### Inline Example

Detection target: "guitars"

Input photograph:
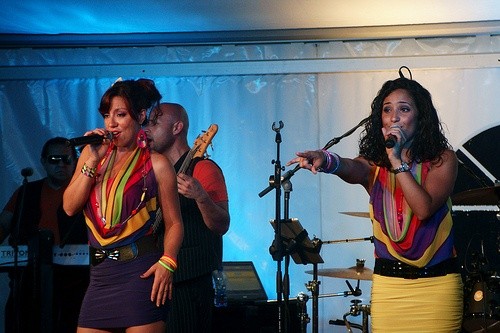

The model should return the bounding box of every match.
[153,123,219,234]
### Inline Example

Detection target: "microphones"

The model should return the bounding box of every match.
[21,167,33,176]
[384,125,401,148]
[68,131,112,147]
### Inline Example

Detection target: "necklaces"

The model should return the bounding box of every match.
[96,136,147,228]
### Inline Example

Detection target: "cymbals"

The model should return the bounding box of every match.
[338,211,370,218]
[451,186,500,205]
[303,265,373,280]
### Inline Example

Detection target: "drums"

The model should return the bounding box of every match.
[462,271,500,333]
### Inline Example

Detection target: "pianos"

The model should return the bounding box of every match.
[0,244,90,333]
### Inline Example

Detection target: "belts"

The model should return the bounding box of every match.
[373,258,459,280]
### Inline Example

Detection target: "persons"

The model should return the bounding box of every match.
[142,102,231,333]
[63,77,183,333]
[287,77,465,333]
[1,137,91,333]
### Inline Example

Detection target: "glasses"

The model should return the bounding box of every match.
[46,154,73,165]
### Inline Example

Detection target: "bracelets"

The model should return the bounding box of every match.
[158,254,178,273]
[80,163,97,178]
[319,149,340,174]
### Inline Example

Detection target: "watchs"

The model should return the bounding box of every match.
[392,161,412,174]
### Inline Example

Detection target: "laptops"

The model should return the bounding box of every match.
[222,261,297,304]
